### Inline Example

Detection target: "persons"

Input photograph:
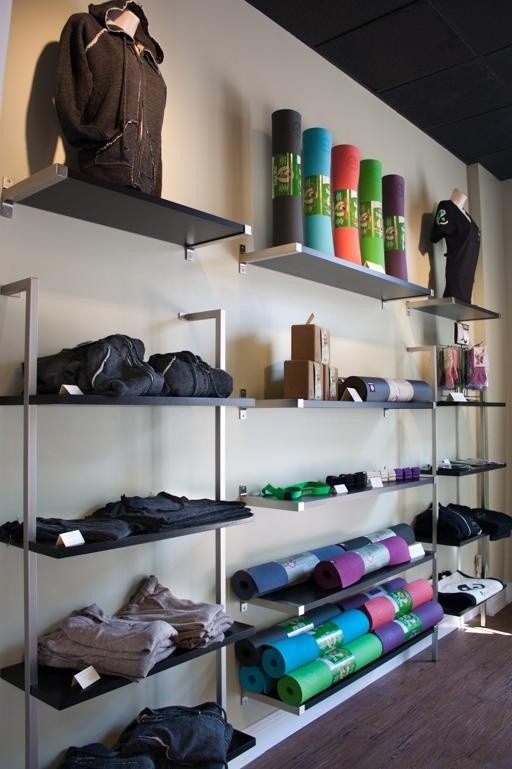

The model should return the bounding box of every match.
[53,2,165,199]
[428,189,480,304]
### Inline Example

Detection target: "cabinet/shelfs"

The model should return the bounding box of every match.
[1,273,256,768]
[405,343,508,664]
[239,388,441,720]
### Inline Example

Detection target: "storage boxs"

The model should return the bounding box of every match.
[283,359,322,402]
[322,365,340,401]
[290,323,331,364]
